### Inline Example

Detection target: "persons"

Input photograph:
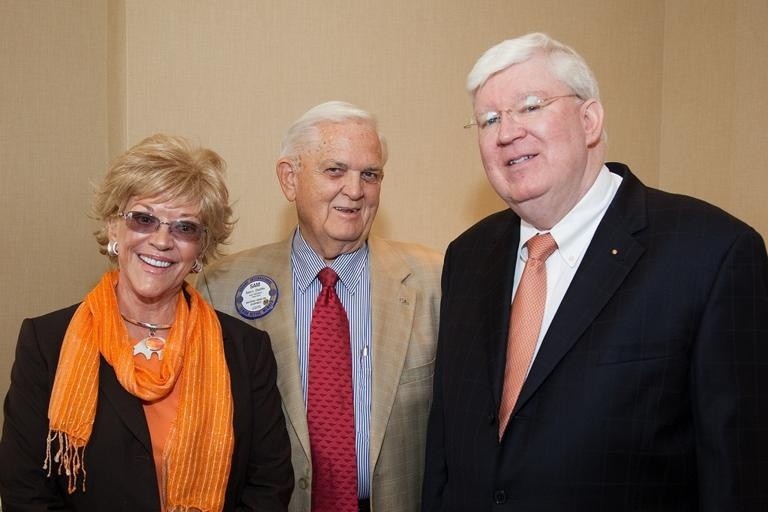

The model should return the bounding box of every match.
[0,133,295,512]
[195,100,445,512]
[421,30,768,512]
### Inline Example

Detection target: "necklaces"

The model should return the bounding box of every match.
[120,310,172,361]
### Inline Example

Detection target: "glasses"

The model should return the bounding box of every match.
[464,94,576,136]
[118,210,206,242]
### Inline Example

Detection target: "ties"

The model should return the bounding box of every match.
[498,234,558,441]
[306,268,359,512]
